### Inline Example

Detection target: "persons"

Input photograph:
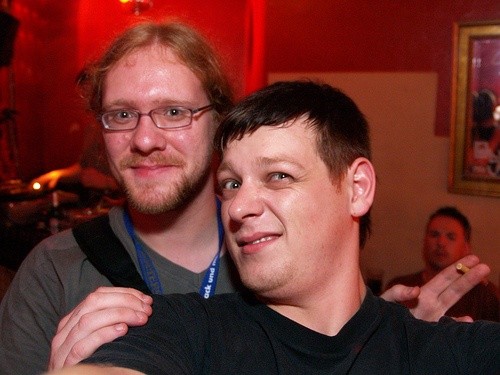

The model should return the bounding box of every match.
[44,80,500,375]
[0,23,492,374]
[385,206,500,323]
[463,87,500,182]
[27,61,129,235]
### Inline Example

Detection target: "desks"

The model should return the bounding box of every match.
[10,189,78,226]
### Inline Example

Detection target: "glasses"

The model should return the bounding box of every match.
[101,103,213,129]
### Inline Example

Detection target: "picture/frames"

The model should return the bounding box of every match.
[446,15,500,199]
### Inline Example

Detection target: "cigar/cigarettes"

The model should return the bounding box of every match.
[456,262,489,287]
